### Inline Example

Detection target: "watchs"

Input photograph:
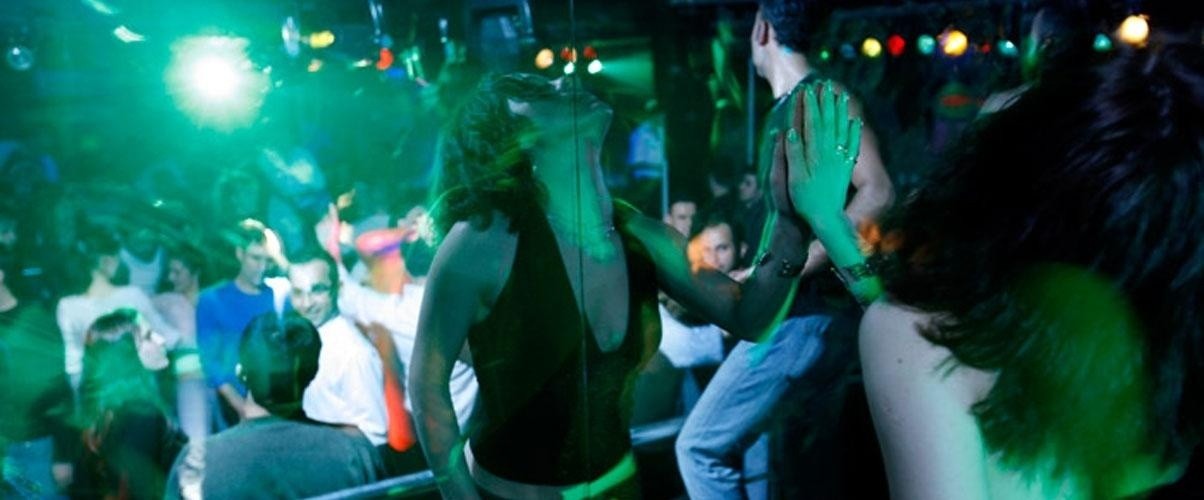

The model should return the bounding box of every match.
[756,249,805,278]
[830,244,892,285]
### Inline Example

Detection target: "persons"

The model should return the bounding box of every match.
[406,67,810,499]
[786,0,1203,500]
[632,154,769,424]
[1,3,480,499]
[673,0,895,499]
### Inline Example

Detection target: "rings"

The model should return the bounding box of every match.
[837,144,848,155]
[846,155,858,164]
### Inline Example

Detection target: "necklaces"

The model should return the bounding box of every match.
[547,210,616,246]
